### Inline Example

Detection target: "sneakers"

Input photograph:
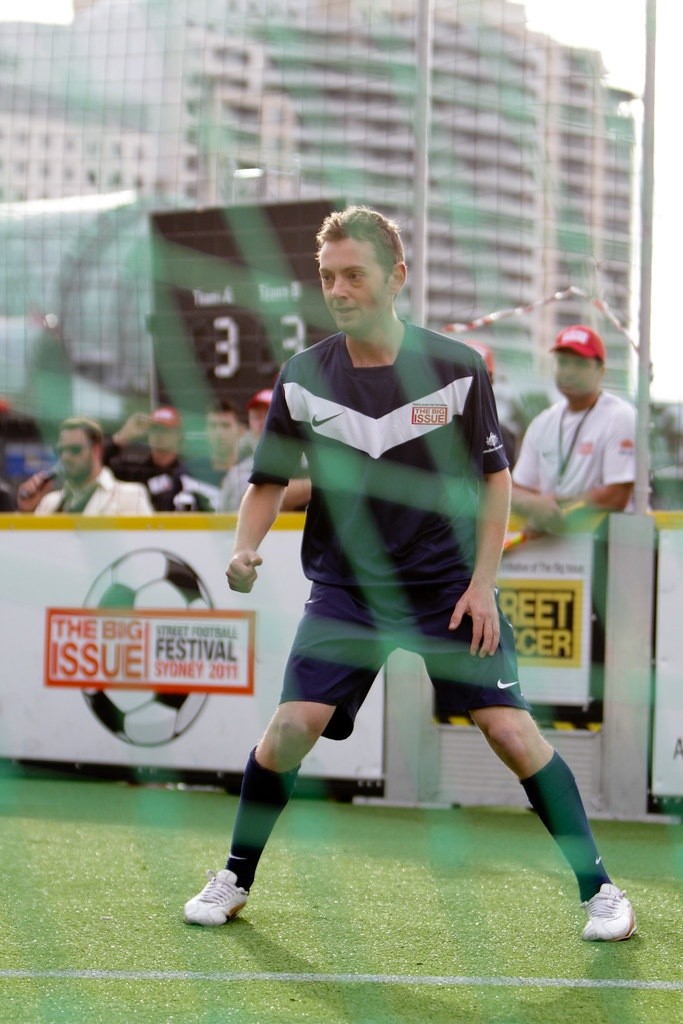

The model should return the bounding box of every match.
[183,870,248,927]
[580,883,637,941]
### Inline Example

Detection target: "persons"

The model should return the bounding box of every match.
[188,398,241,511]
[17,419,152,513]
[510,326,653,533]
[100,408,200,511]
[464,341,516,470]
[221,391,311,511]
[183,209,637,941]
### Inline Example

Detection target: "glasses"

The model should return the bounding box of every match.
[52,443,93,456]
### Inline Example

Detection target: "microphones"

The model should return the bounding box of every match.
[20,463,66,501]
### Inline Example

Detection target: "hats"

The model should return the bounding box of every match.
[467,340,494,373]
[248,390,272,407]
[150,408,180,428]
[549,326,605,364]
[113,412,150,445]
[7,448,57,474]
